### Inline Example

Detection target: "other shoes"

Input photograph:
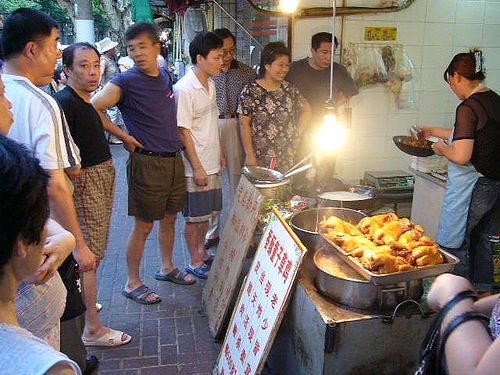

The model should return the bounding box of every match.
[204,236,219,250]
[108,138,124,144]
[83,355,98,375]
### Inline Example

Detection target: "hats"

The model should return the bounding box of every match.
[95,37,119,55]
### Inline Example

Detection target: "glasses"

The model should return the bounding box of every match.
[222,47,237,57]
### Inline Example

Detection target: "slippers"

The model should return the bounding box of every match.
[185,252,215,279]
[121,283,161,305]
[154,267,197,286]
[81,328,132,347]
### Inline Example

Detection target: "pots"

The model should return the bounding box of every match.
[254,176,293,202]
[288,207,368,255]
[312,245,424,315]
[316,184,378,212]
[392,135,435,157]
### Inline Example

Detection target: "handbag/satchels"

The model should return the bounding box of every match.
[354,47,415,90]
[411,289,491,375]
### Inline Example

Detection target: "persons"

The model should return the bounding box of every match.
[238,42,312,174]
[428,273,500,375]
[0,8,99,375]
[0,133,81,375]
[90,22,196,303]
[172,31,224,278]
[205,28,258,248]
[417,47,500,290]
[54,42,132,346]
[284,32,359,184]
[42,70,67,94]
[0,74,76,350]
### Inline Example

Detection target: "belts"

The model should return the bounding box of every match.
[219,112,239,119]
[134,145,182,158]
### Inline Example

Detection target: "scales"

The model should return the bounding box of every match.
[365,169,415,192]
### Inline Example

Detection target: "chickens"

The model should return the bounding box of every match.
[319,213,444,271]
